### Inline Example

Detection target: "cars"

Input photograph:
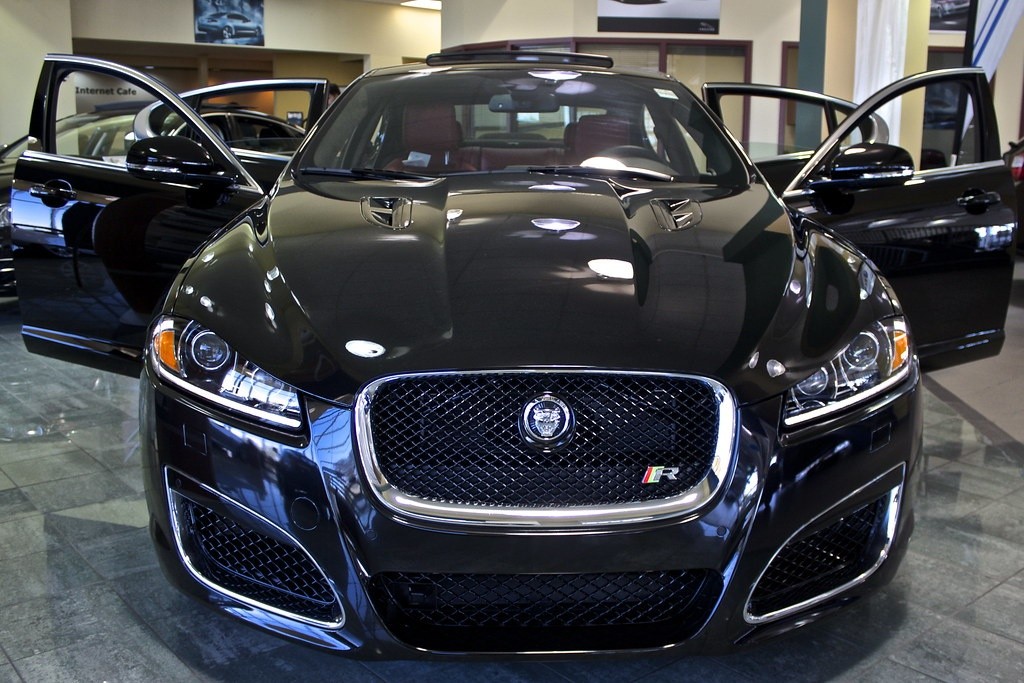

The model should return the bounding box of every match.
[999,136,1024,210]
[9,51,1020,659]
[0,98,319,306]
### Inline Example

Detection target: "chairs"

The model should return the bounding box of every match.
[379,96,475,172]
[574,113,640,164]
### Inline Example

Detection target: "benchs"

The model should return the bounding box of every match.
[453,121,577,166]
